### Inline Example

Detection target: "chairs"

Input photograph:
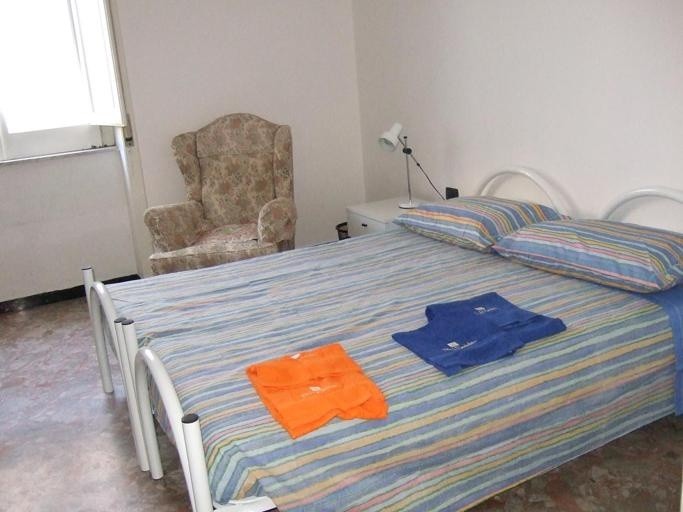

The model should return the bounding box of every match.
[144,113,296,275]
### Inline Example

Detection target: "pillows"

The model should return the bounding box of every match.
[392,195,573,254]
[490,218,683,294]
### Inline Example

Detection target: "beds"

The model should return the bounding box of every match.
[80,167,683,512]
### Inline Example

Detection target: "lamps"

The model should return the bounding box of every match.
[380,122,446,209]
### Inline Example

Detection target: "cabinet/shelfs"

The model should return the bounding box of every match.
[346,196,431,239]
